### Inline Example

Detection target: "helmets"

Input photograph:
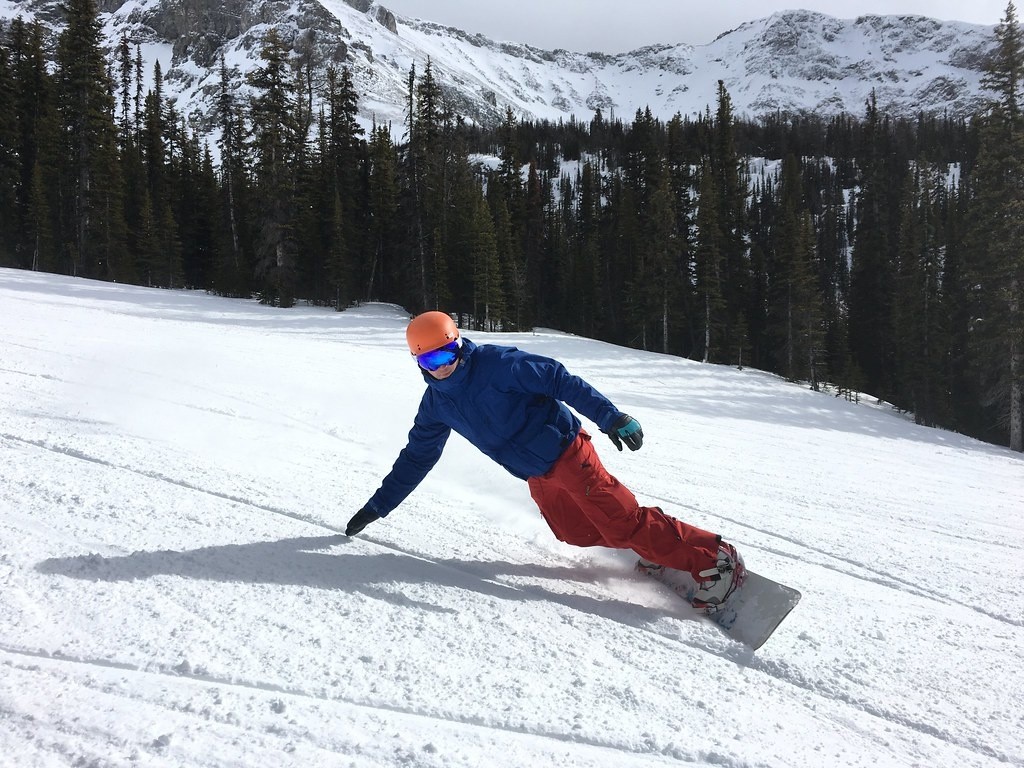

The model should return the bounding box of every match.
[407,311,459,357]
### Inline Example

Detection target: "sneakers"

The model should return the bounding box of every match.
[633,557,668,577]
[691,543,744,614]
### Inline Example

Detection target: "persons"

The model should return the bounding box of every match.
[345,311,747,614]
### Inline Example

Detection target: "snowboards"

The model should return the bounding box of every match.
[633,539,802,651]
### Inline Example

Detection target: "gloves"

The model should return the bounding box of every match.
[608,414,644,452]
[345,506,380,536]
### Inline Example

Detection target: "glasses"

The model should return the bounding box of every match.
[417,342,460,371]
